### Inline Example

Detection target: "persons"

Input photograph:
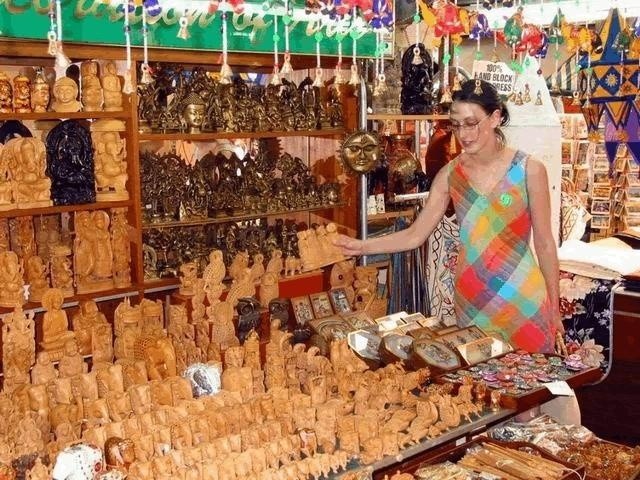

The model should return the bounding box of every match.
[331,77,564,424]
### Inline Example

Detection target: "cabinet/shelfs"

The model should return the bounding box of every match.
[1,38,360,373]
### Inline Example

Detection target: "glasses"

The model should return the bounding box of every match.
[447,113,492,130]
